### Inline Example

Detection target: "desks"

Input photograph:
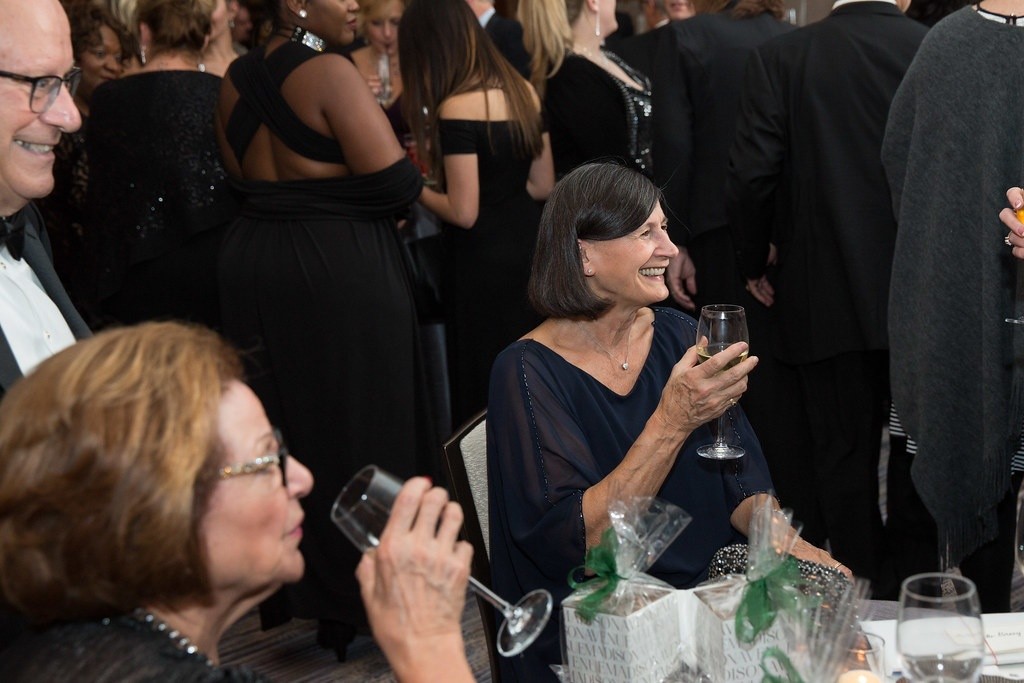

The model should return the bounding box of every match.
[858,601,1022,683]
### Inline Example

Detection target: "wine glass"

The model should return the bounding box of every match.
[695,303,749,459]
[332,464,553,658]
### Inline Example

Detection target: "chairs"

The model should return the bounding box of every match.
[444,402,566,683]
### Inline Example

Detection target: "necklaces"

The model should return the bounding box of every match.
[590,329,632,370]
[139,607,212,666]
[576,44,609,66]
[275,23,325,52]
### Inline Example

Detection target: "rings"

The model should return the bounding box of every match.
[834,562,841,569]
[1005,229,1013,245]
[730,398,738,407]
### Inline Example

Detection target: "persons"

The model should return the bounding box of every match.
[0,322,478,683]
[0,0,97,390]
[33,0,1024,660]
[485,162,854,683]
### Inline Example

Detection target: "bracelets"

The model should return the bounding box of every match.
[758,506,788,523]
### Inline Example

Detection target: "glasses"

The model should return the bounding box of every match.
[0,65,82,113]
[198,427,289,486]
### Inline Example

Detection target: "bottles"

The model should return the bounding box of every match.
[403,133,428,185]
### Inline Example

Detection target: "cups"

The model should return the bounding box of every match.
[832,632,885,682]
[894,572,985,683]
[369,54,396,99]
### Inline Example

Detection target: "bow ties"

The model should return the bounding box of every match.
[0,208,25,261]
